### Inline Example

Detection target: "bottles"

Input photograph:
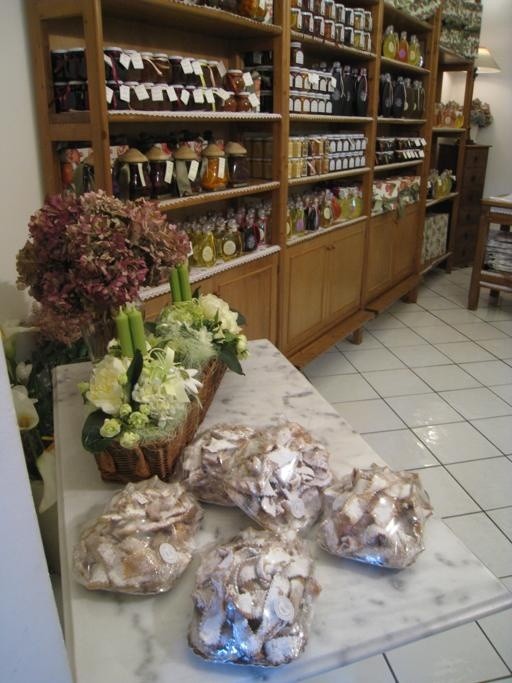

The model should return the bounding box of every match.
[382,24,422,66]
[380,73,425,117]
[436,102,463,127]
[430,168,453,196]
[249,187,362,240]
[176,208,269,268]
[310,62,369,117]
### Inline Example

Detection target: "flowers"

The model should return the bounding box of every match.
[78,294,248,454]
[470,99,493,129]
[16,190,192,326]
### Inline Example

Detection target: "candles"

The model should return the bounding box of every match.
[50,336,512,682]
[128,300,148,357]
[169,265,182,303]
[178,262,192,301]
[114,304,134,358]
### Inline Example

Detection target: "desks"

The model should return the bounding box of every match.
[468,194,512,311]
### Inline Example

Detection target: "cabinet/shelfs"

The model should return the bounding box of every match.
[27,0,475,373]
[439,142,492,270]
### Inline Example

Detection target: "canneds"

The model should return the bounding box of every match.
[52,48,254,114]
[241,132,366,179]
[247,42,334,114]
[289,0,373,53]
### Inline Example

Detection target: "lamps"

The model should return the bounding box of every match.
[474,46,501,79]
